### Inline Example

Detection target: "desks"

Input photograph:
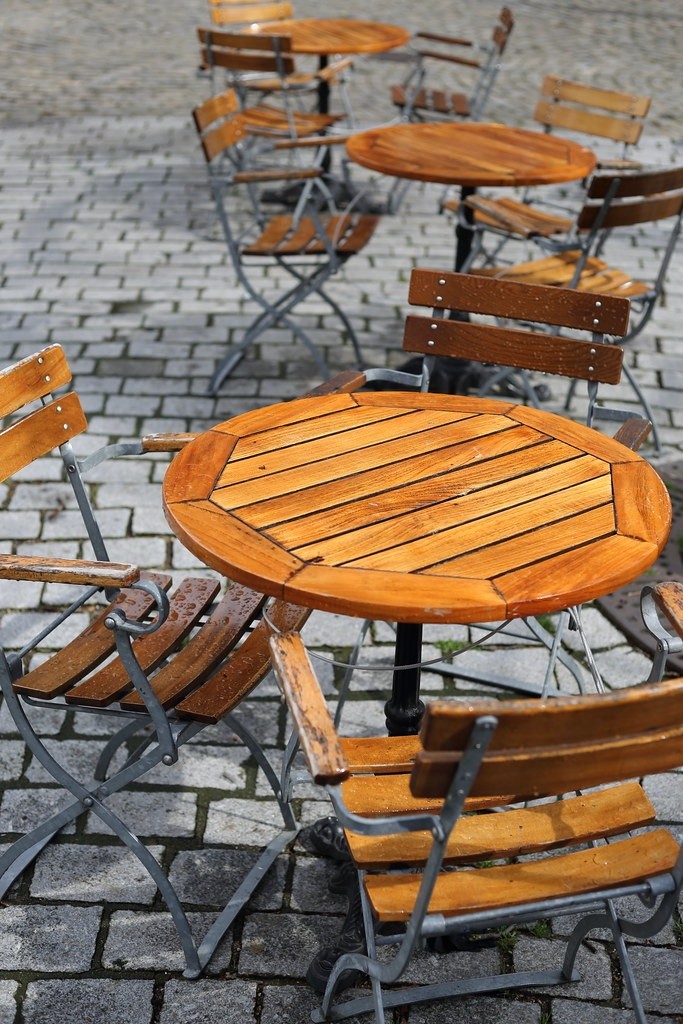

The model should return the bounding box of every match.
[346,122,599,401]
[242,16,409,216]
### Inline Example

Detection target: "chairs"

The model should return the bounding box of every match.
[191,0,683,458]
[267,580,683,1024]
[286,269,653,698]
[0,343,316,980]
